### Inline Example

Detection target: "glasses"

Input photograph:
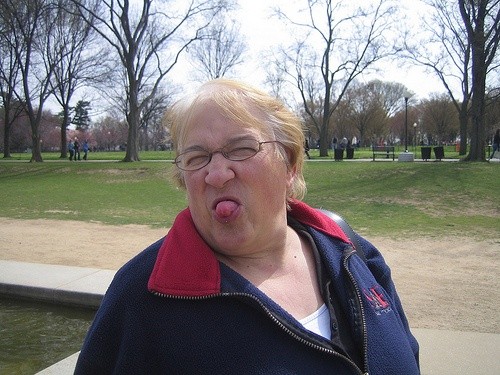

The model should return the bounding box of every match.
[172,139,280,171]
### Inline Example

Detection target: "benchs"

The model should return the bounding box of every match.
[372,145,395,161]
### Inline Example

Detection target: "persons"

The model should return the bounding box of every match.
[486,129,500,162]
[68,139,88,160]
[73,80,420,375]
[304,136,388,160]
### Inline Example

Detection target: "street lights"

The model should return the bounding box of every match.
[413,123,418,153]
[403,92,412,153]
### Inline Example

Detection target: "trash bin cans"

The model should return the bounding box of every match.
[346,147,354,158]
[335,148,345,159]
[434,146,444,158]
[421,147,431,159]
